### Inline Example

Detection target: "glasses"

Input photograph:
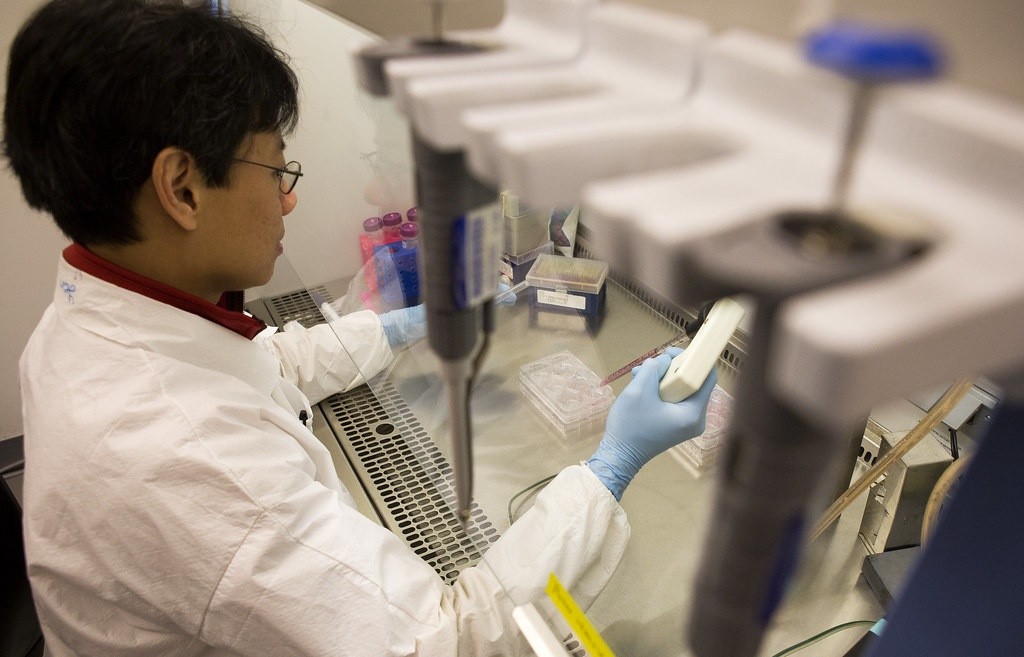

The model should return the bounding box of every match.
[232,156,304,195]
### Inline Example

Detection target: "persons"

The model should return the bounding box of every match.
[0,0,719,657]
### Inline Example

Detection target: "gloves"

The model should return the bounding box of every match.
[585,345,721,502]
[379,280,518,346]
